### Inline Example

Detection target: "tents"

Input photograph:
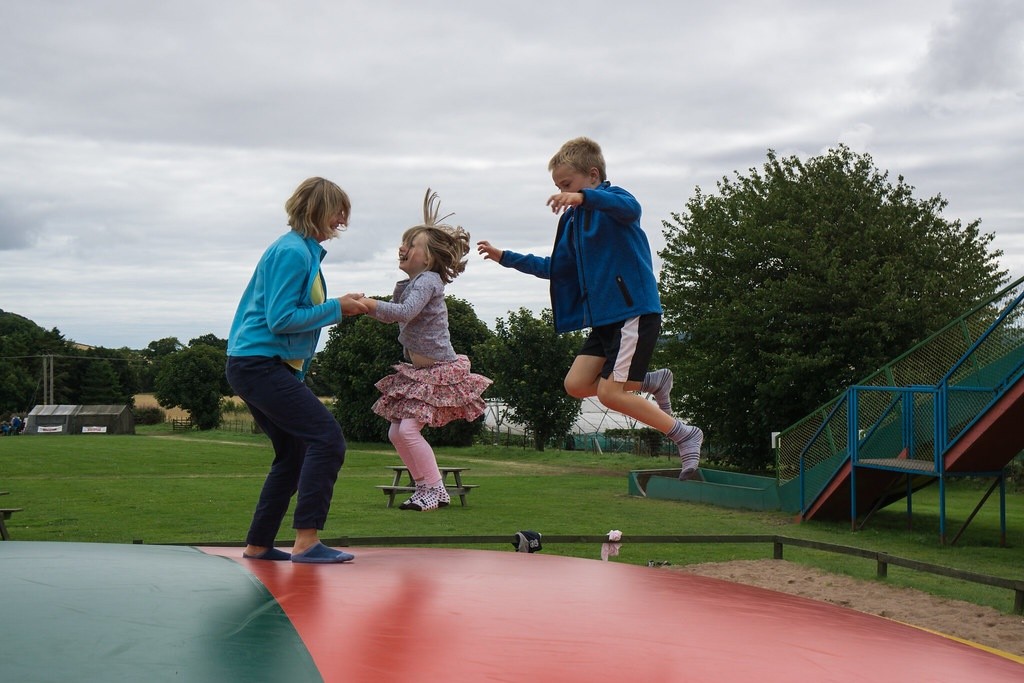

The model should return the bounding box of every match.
[21,405,135,434]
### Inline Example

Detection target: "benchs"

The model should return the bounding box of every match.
[375,485,415,490]
[444,484,479,488]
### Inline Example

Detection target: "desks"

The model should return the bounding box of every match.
[387,466,467,509]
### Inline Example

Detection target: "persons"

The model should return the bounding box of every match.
[0,415,25,436]
[226,177,369,562]
[477,138,703,480]
[352,189,493,511]
